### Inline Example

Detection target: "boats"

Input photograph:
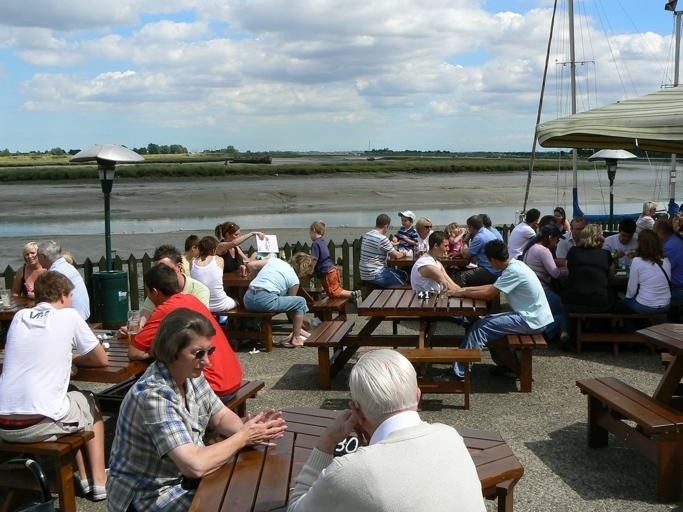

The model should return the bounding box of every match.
[227,152,273,166]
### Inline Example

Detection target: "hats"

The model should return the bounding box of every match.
[541,224,566,240]
[397,209,416,226]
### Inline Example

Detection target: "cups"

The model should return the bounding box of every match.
[0,289,12,307]
[126,310,141,334]
[439,281,449,300]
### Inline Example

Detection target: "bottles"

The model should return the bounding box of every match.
[102,333,110,349]
[610,249,619,270]
[97,334,102,343]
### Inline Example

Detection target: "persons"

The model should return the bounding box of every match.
[0,270,108,501]
[127,262,244,404]
[105,306,288,512]
[0,199,683,378]
[289,349,488,512]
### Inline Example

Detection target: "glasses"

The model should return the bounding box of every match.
[191,346,216,360]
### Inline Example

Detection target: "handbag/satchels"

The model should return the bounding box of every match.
[657,262,679,308]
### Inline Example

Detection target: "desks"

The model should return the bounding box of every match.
[387,252,471,274]
[221,267,317,351]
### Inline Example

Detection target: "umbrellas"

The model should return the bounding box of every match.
[535,85,683,207]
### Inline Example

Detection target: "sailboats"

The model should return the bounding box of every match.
[365,140,376,161]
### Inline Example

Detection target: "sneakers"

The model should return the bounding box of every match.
[490,364,518,377]
[433,369,471,384]
[79,468,109,503]
